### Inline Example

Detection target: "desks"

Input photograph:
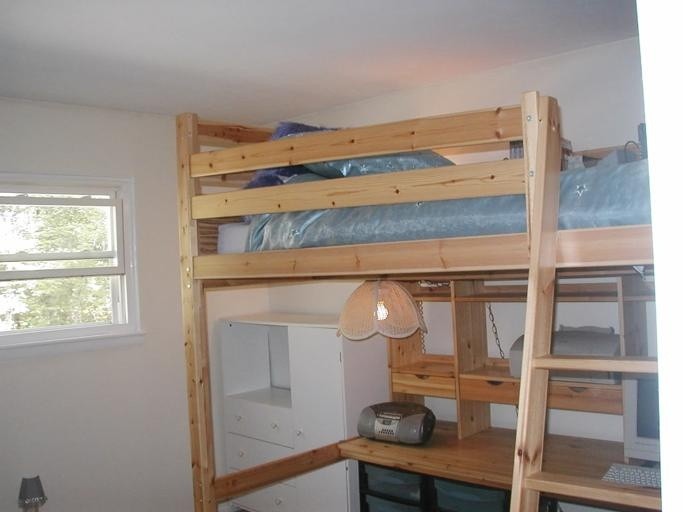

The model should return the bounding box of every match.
[336,409,660,512]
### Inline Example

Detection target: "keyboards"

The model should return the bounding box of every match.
[601,461,661,490]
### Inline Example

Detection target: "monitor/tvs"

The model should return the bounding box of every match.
[621,377,660,466]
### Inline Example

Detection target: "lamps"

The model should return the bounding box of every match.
[338,273,428,342]
[18,475,48,512]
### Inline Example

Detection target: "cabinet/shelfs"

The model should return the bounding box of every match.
[374,278,660,439]
[219,310,368,511]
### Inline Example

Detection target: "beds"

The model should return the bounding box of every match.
[175,91,660,511]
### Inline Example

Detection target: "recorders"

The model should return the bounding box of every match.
[356,398,435,445]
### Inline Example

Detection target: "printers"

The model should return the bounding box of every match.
[507,323,619,385]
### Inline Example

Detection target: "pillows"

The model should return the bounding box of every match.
[279,130,457,178]
